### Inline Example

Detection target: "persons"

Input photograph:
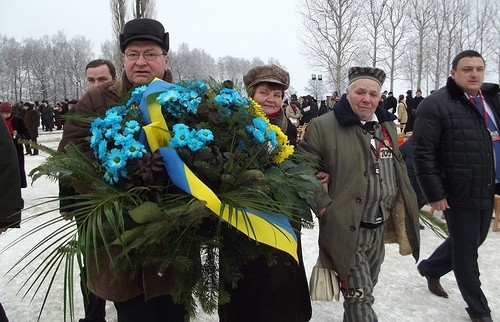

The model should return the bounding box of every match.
[295,65,421,322]
[217,64,312,321]
[410,49,499,322]
[60,59,133,322]
[222,79,235,90]
[0,112,29,322]
[0,102,32,210]
[58,18,204,322]
[10,98,78,155]
[284,89,436,152]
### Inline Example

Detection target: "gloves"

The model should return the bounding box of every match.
[60,211,74,222]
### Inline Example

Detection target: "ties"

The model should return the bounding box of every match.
[472,96,500,184]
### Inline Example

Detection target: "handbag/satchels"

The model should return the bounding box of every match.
[309,260,341,304]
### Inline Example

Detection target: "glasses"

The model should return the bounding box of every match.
[123,51,164,61]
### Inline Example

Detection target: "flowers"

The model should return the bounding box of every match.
[0,77,329,322]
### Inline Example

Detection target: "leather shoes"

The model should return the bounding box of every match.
[464,307,494,322]
[416,260,448,299]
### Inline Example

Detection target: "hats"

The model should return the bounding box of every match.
[243,64,291,90]
[399,94,405,100]
[347,67,387,87]
[118,17,170,54]
[24,102,31,108]
[406,90,412,94]
[0,101,13,114]
[283,94,315,105]
